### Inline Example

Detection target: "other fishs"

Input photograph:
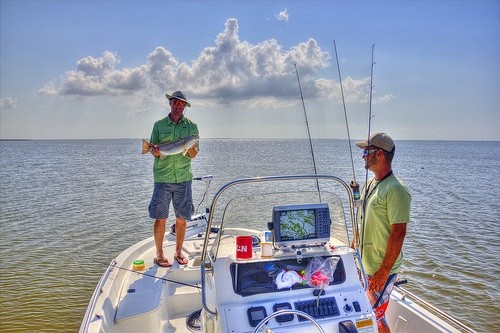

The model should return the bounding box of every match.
[142,135,200,160]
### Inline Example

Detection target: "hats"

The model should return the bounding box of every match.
[356,133,395,153]
[165,91,192,108]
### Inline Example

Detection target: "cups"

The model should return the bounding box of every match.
[262,242,273,258]
[265,232,272,242]
[236,236,253,259]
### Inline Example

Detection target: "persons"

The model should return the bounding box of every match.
[356,131,412,333]
[148,90,199,267]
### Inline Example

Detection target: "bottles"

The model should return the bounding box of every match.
[133,259,146,270]
[265,263,280,278]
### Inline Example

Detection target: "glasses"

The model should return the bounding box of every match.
[363,149,378,156]
[171,100,185,106]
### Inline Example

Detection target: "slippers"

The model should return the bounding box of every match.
[174,255,187,265]
[154,258,172,267]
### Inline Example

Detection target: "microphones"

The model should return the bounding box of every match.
[201,175,213,179]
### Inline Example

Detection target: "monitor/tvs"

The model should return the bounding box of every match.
[271,204,330,249]
